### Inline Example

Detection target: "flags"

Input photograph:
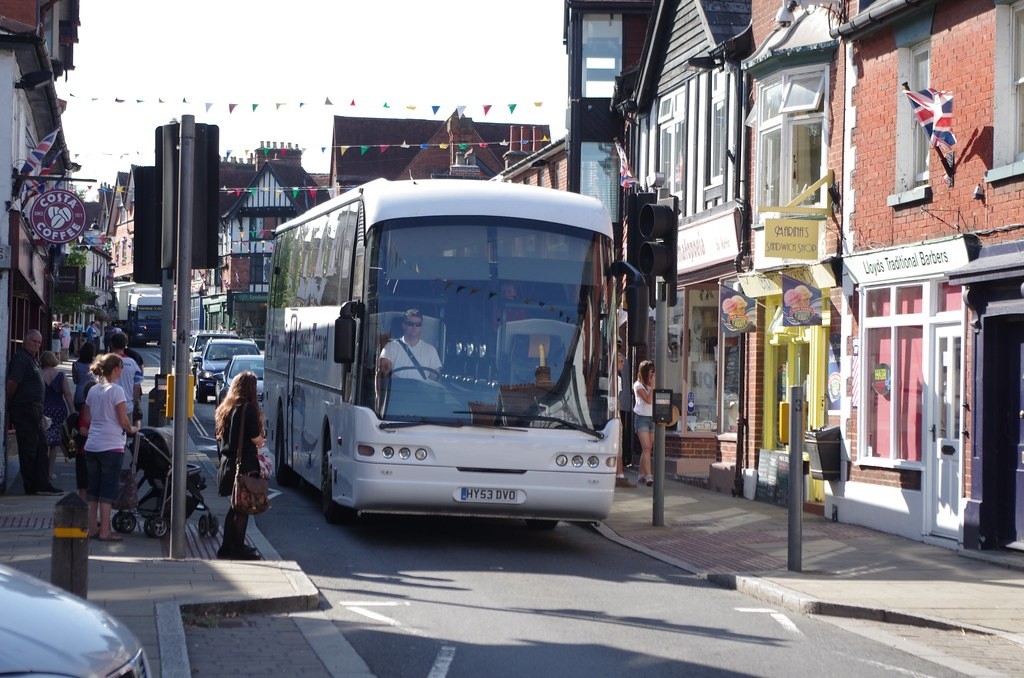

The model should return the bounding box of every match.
[901,88,956,151]
[611,138,642,188]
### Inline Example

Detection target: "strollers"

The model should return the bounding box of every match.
[110,424,221,541]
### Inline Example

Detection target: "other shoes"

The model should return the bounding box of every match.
[217,544,261,560]
[615,477,637,489]
[35,482,65,496]
[646,480,654,487]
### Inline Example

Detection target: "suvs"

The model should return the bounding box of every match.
[191,336,261,405]
[189,328,240,372]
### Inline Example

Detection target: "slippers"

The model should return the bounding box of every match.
[98,531,122,543]
[88,531,100,539]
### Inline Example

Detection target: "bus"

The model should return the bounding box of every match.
[261,178,653,534]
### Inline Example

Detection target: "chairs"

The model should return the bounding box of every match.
[390,316,406,342]
[511,333,562,368]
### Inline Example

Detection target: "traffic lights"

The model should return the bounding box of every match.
[636,196,679,283]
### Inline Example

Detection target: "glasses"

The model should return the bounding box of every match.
[403,321,422,328]
[649,369,656,373]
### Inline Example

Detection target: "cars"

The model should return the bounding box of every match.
[211,355,267,417]
[1,561,152,678]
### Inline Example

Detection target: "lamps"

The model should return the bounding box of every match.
[689,56,723,70]
[531,158,549,168]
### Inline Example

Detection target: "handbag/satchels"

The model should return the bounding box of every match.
[231,472,271,515]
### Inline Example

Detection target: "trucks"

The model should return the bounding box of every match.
[127,287,164,346]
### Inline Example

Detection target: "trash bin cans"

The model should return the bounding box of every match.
[148,374,172,427]
[805,426,842,479]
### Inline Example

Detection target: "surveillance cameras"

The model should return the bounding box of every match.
[776,7,795,28]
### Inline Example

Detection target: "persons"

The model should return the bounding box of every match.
[7,329,67,497]
[84,353,138,543]
[380,307,444,382]
[35,348,77,481]
[616,353,637,488]
[70,342,95,413]
[102,316,145,426]
[212,369,266,560]
[87,320,101,354]
[77,381,97,502]
[52,320,71,363]
[630,360,656,485]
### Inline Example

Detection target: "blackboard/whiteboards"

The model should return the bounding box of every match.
[756,450,789,507]
